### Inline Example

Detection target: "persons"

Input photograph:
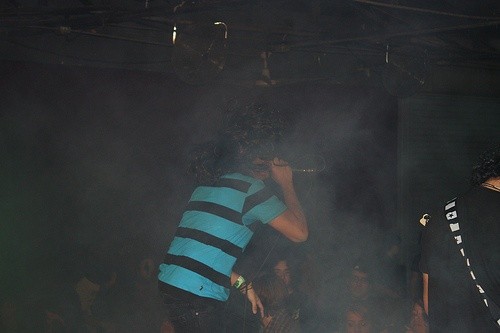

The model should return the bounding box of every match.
[419,150,500,333]
[0,247,428,333]
[157,137,309,333]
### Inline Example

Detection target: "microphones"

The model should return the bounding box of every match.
[291,166,320,176]
